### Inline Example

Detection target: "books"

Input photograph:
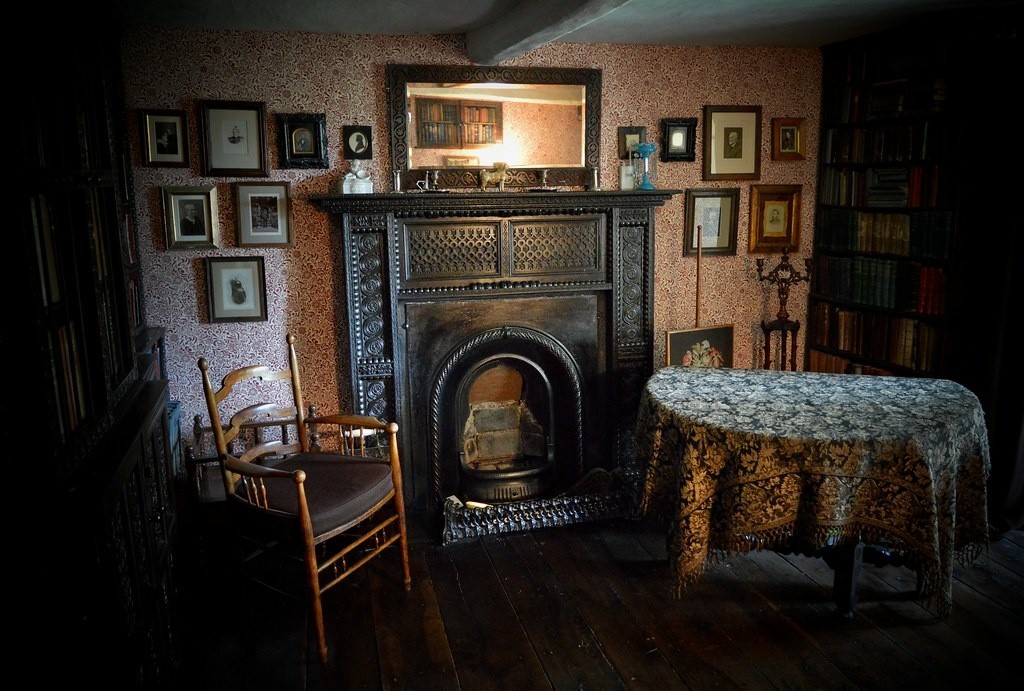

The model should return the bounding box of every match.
[129,279,141,332]
[47,326,88,445]
[28,195,61,308]
[419,104,497,143]
[812,30,990,375]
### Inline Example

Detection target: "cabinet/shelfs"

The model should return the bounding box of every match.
[0,0,182,691]
[409,94,504,150]
[804,32,953,378]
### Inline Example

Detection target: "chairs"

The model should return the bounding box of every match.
[197,331,413,662]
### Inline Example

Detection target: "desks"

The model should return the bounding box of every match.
[617,362,992,633]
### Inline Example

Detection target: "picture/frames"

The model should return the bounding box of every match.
[140,107,192,167]
[195,99,271,178]
[665,322,735,369]
[204,256,268,324]
[276,112,330,170]
[159,185,220,250]
[771,118,808,161]
[702,105,762,181]
[342,125,373,160]
[660,118,698,162]
[683,187,740,257]
[746,184,803,254]
[618,126,650,159]
[234,181,295,247]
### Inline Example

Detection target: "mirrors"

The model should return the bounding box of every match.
[385,64,603,191]
